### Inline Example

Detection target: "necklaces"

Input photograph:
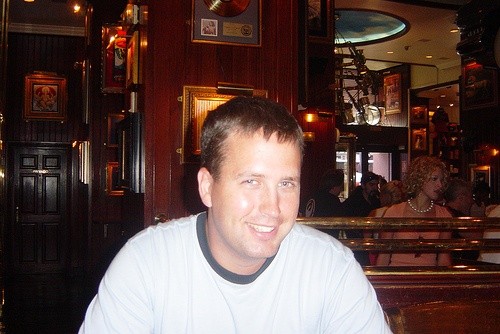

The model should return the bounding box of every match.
[408,198,433,213]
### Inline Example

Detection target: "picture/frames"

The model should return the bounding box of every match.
[23,19,139,198]
[383,71,402,114]
[191,0,263,49]
[471,164,491,201]
[307,0,334,46]
[461,57,498,112]
[179,85,268,165]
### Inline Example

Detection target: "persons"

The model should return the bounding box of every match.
[328,155,500,266]
[78,95,394,334]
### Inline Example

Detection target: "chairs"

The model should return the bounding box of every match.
[389,299,500,334]
